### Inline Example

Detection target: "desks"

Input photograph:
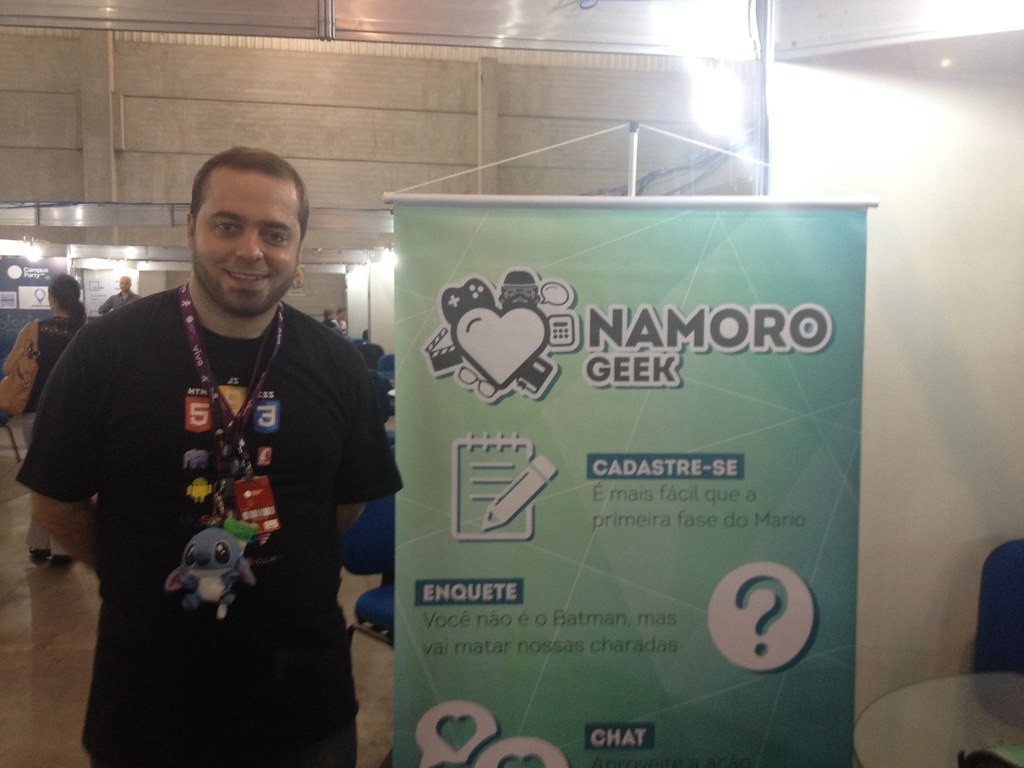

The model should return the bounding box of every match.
[850,672,1024,768]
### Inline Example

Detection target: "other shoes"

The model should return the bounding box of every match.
[29,546,53,560]
[49,554,75,567]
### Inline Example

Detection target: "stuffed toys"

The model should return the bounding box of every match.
[165,526,256,612]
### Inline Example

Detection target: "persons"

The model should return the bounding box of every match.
[359,329,369,345]
[15,145,403,768]
[98,276,142,315]
[331,306,347,336]
[320,308,341,333]
[3,274,87,568]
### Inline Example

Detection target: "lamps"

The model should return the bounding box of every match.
[553,0,598,9]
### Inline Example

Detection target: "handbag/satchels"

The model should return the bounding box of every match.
[1,320,44,419]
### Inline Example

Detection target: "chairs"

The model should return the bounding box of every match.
[974,539,1024,671]
